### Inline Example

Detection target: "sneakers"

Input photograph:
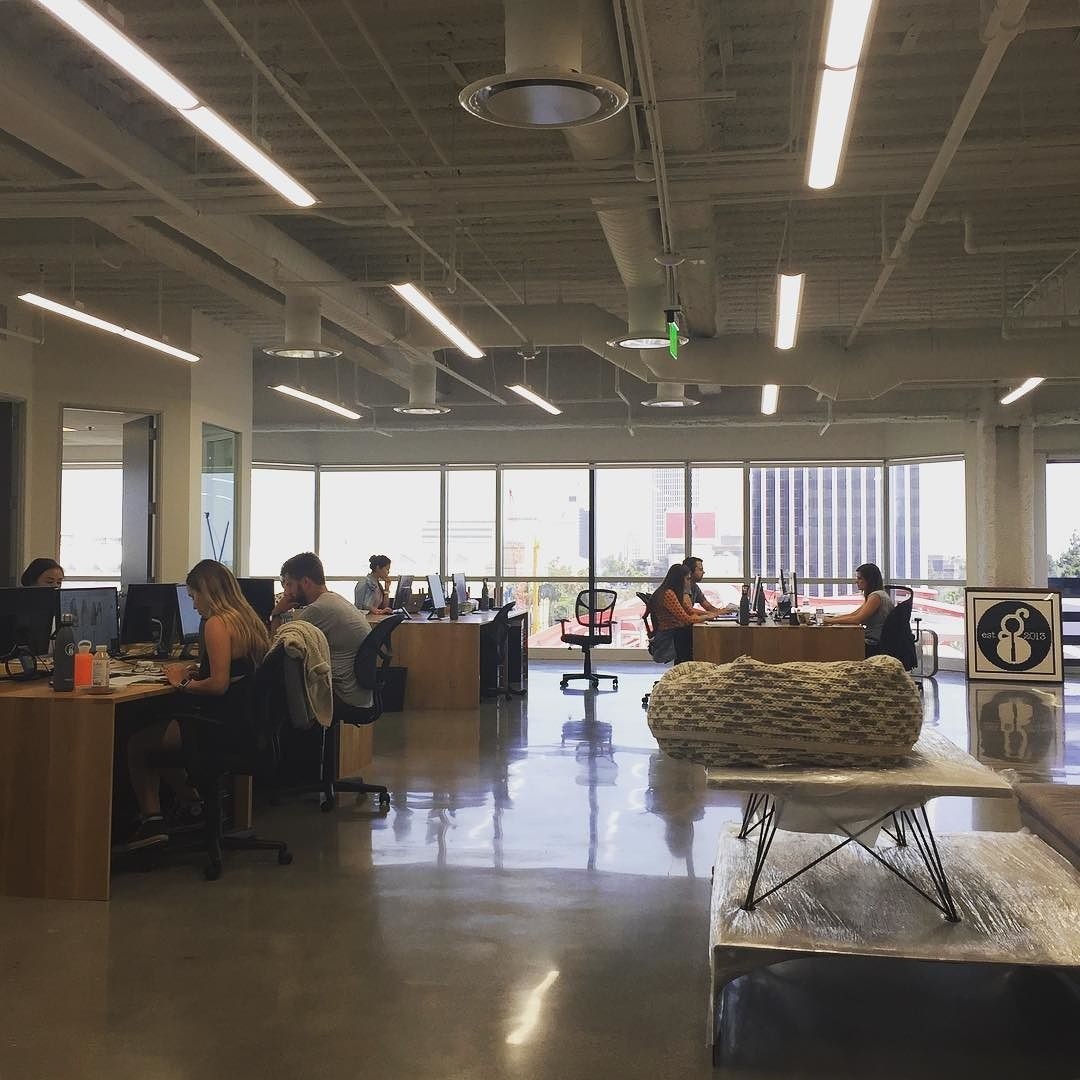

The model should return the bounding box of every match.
[111,815,168,856]
[165,807,207,833]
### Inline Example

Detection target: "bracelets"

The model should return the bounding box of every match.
[384,591,390,594]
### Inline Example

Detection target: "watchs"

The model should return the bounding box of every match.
[178,678,192,693]
[270,614,280,622]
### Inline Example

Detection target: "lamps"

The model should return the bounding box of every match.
[17,268,1052,421]
[178,106,323,209]
[27,0,206,110]
[804,66,862,189]
[817,1,881,69]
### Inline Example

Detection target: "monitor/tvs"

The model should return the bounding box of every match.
[236,577,274,622]
[0,586,59,680]
[772,569,787,612]
[452,572,467,608]
[119,583,178,660]
[393,576,413,620]
[782,572,799,619]
[55,586,119,654]
[427,574,447,620]
[174,584,205,661]
[751,574,770,625]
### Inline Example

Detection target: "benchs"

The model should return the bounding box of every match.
[1015,780,1080,872]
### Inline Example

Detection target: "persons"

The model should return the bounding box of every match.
[354,554,394,615]
[112,558,278,855]
[678,557,733,650]
[649,564,719,664]
[19,557,66,655]
[821,563,896,663]
[267,552,382,765]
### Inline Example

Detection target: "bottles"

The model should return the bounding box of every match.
[94,645,110,687]
[450,583,458,620]
[758,587,765,617]
[482,581,489,611]
[51,614,77,692]
[740,585,750,625]
[802,601,810,623]
[74,640,94,688]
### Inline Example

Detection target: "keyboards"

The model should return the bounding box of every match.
[393,608,404,613]
[719,612,738,617]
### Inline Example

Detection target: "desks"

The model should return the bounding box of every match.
[706,829,1080,1065]
[365,609,529,709]
[692,618,865,665]
[704,722,1010,926]
[0,656,374,902]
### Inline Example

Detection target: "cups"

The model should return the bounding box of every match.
[778,597,791,618]
[816,613,824,626]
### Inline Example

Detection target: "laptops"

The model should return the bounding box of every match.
[406,593,426,613]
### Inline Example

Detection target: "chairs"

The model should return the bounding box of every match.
[637,592,692,704]
[560,589,618,689]
[164,620,331,881]
[481,601,526,700]
[271,614,406,813]
[866,585,924,688]
